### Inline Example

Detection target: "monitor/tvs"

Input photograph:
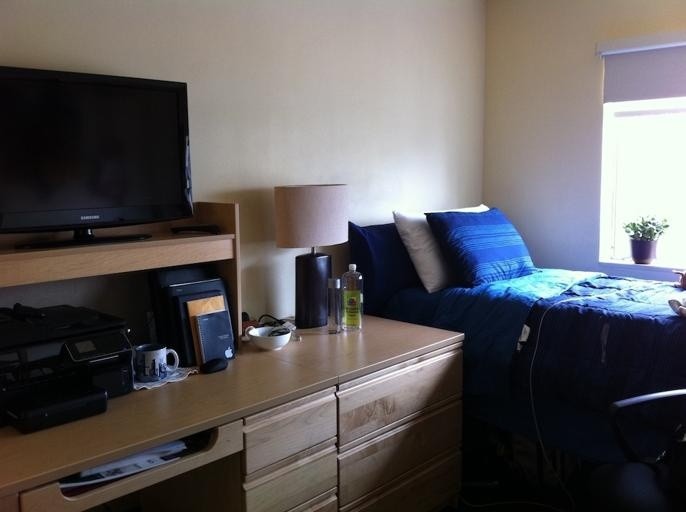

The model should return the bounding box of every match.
[0,64,194,251]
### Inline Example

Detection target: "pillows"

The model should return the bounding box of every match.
[391,204,495,294]
[424,205,544,287]
[346,219,423,292]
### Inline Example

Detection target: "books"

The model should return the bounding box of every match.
[55,440,191,488]
[142,264,236,364]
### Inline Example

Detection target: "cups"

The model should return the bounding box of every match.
[136,344,180,382]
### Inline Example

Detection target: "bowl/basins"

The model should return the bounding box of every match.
[248,327,291,350]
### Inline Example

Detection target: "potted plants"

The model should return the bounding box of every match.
[621,216,672,265]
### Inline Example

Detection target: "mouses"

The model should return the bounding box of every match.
[198,357,229,374]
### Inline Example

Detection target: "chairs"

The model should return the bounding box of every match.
[567,387,686,512]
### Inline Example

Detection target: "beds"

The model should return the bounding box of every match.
[312,241,685,512]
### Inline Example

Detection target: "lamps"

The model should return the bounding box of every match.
[273,182,352,331]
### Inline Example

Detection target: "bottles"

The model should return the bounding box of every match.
[328,279,341,333]
[341,263,364,330]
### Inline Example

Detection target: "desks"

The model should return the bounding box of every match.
[0,197,469,512]
